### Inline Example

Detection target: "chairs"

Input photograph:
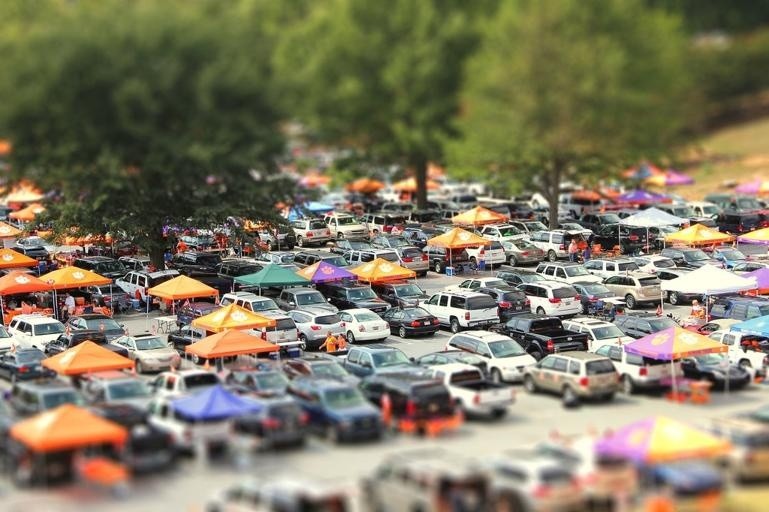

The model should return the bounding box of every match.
[476,260,485,277]
[2,297,114,326]
[464,266,471,276]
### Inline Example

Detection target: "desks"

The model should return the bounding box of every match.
[659,375,712,405]
[278,341,306,360]
[154,315,177,334]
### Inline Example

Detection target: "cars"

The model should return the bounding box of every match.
[0,180,769,512]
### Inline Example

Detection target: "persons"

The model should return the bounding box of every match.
[318,331,337,352]
[336,333,346,351]
[60,292,75,321]
[470,258,480,271]
[691,299,701,317]
[581,247,590,263]
[176,238,187,253]
[568,239,578,261]
